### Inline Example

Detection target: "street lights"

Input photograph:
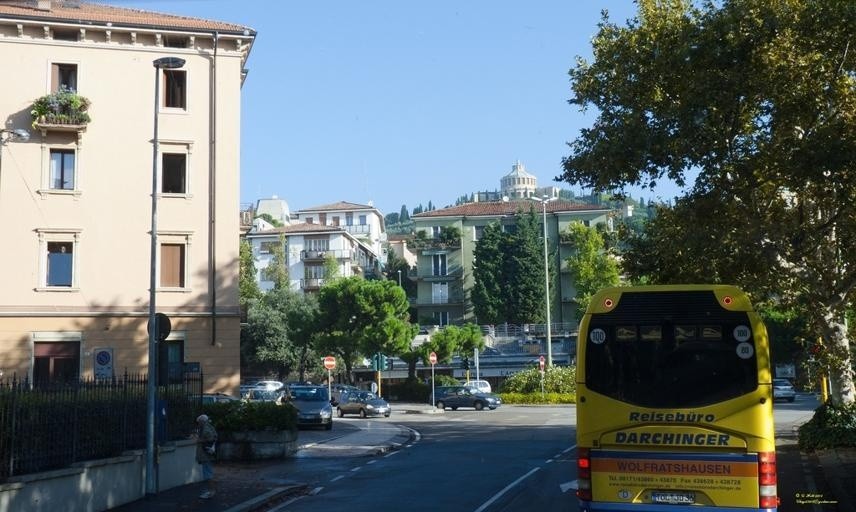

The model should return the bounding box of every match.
[542,195,557,369]
[145,58,187,497]
[397,269,402,287]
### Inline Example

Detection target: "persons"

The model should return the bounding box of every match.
[194,413,218,500]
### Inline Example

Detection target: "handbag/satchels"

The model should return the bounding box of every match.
[203,442,216,455]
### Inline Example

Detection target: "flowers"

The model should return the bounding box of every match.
[31,84,92,129]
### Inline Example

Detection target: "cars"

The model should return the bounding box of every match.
[286,383,332,431]
[804,382,816,392]
[771,379,795,404]
[326,383,391,419]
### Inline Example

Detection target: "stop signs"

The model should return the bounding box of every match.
[324,357,335,370]
[429,353,437,364]
[540,355,546,370]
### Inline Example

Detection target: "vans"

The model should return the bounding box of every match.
[463,380,492,393]
[429,385,503,411]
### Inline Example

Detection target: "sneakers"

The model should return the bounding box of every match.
[200,491,216,499]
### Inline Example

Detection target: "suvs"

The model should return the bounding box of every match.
[178,391,238,407]
[241,380,289,404]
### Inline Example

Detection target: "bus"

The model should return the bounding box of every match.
[576,283,778,512]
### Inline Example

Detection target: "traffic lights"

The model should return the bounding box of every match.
[463,358,470,369]
[372,353,388,371]
